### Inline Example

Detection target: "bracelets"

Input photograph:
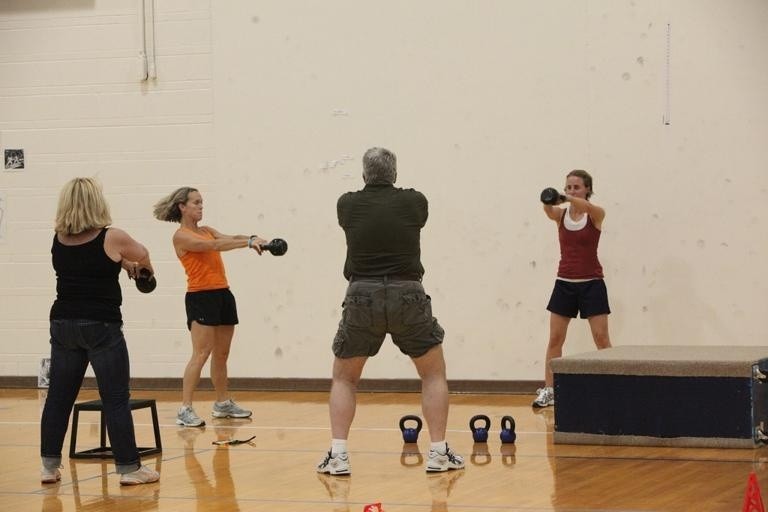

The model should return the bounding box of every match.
[248,239,253,248]
[250,235,258,240]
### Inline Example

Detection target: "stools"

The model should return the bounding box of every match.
[70,399,162,459]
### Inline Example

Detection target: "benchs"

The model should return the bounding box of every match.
[549,346,768,448]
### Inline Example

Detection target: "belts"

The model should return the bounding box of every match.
[349,274,420,281]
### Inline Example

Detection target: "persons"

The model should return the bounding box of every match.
[530,170,612,408]
[315,147,464,474]
[152,187,269,427]
[39,177,159,485]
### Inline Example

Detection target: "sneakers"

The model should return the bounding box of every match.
[426,442,465,472]
[211,400,252,418]
[316,446,352,476]
[176,405,206,427]
[40,464,64,483]
[532,386,555,408]
[120,464,160,484]
